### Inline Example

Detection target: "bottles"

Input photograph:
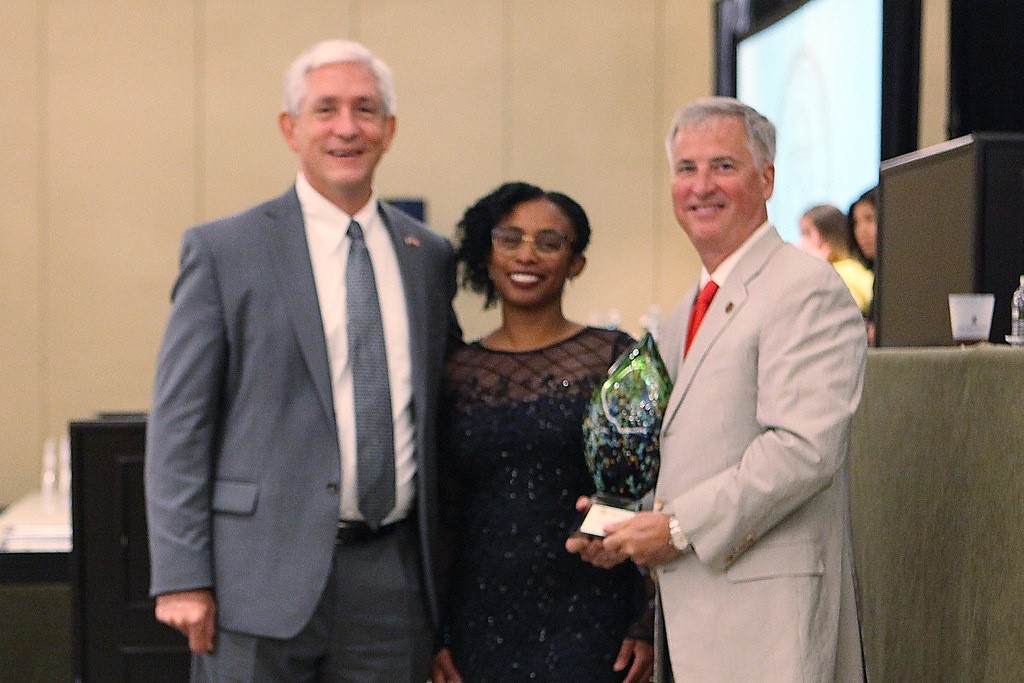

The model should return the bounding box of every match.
[1011,275,1024,346]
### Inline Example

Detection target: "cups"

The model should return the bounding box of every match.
[948,293,995,346]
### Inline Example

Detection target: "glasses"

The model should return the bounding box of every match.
[490,227,576,256]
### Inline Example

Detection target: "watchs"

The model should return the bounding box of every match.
[669,515,691,554]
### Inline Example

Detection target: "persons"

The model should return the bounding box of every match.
[565,98,867,683]
[800,185,880,346]
[141,41,461,683]
[434,182,653,683]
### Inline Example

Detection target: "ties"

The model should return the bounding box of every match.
[683,280,719,358]
[346,221,396,534]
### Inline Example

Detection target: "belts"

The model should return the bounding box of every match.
[337,522,401,541]
